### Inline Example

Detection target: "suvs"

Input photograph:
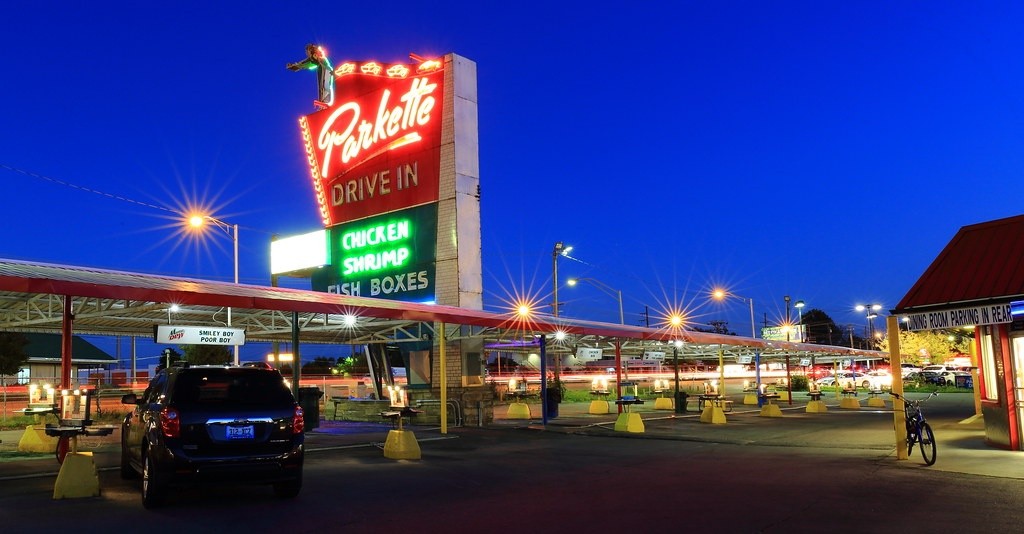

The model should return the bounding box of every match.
[121,360,305,508]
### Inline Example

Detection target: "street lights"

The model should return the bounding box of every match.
[713,288,756,338]
[856,303,882,370]
[568,275,624,325]
[188,215,239,284]
[553,241,574,383]
[794,299,805,343]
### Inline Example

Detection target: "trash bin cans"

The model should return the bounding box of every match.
[298,387,319,431]
[926,376,945,386]
[955,376,973,389]
[675,392,686,414]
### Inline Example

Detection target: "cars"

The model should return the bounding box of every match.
[815,370,894,389]
[901,362,967,385]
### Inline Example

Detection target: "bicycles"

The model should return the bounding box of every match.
[889,390,942,466]
